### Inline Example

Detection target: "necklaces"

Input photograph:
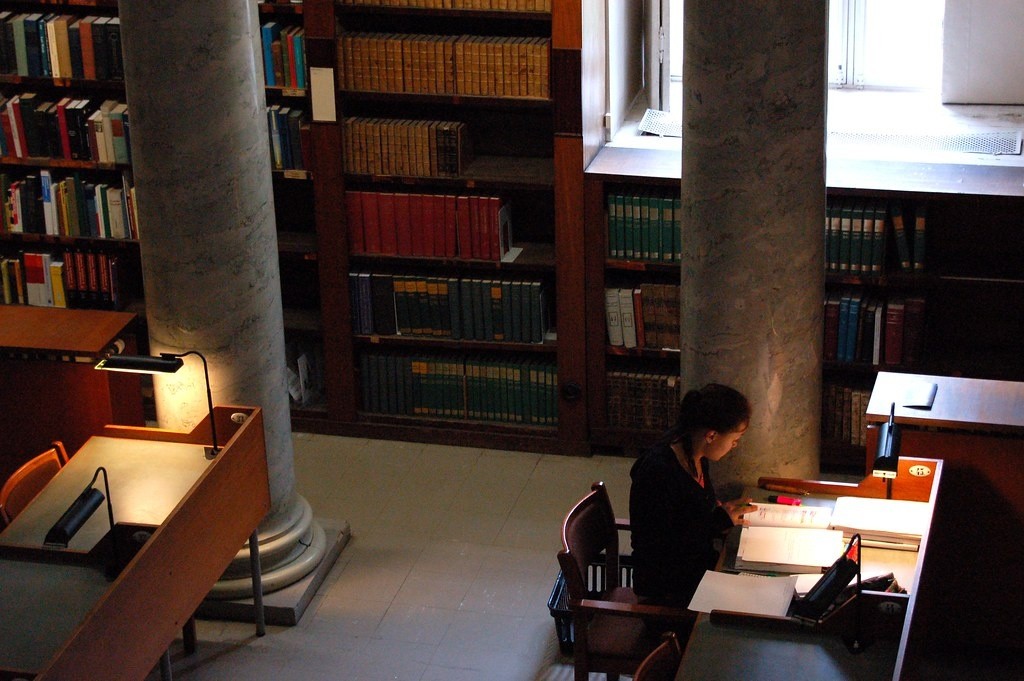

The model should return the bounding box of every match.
[673,444,704,480]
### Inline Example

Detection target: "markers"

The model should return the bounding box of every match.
[768,495,801,506]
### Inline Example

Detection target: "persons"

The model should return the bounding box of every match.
[630,383,758,610]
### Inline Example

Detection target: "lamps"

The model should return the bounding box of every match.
[46,465,161,582]
[100,350,218,456]
[791,532,867,655]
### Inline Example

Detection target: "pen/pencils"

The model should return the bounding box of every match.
[739,503,753,506]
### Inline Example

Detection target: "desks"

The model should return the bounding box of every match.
[0,304,146,489]
[865,371,1024,512]
[0,406,272,681]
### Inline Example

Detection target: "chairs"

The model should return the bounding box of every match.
[557,480,728,681]
[633,631,681,681]
[0,441,71,527]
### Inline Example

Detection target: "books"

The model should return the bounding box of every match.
[822,381,871,447]
[735,496,929,574]
[0,168,139,239]
[0,90,131,164]
[602,277,681,348]
[823,284,925,366]
[348,269,553,344]
[361,349,560,425]
[344,186,516,261]
[608,186,681,262]
[605,359,680,430]
[824,197,927,273]
[0,247,125,365]
[332,0,551,98]
[0,6,124,81]
[260,20,312,170]
[341,107,476,178]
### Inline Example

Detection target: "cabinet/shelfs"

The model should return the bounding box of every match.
[0,0,1024,472]
